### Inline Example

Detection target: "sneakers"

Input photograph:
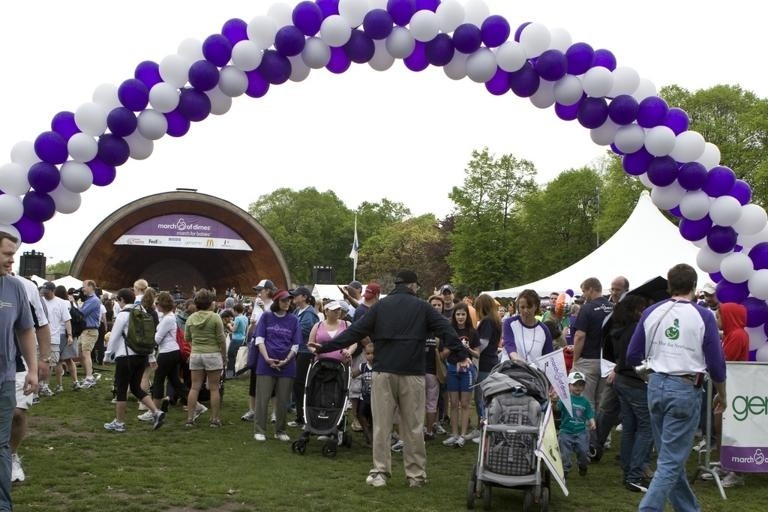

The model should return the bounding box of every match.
[240,409,303,441]
[351,421,363,431]
[625,477,650,493]
[33,372,102,404]
[366,473,388,488]
[578,463,588,476]
[391,423,481,453]
[10,453,25,482]
[137,396,222,430]
[104,418,125,432]
[692,435,744,488]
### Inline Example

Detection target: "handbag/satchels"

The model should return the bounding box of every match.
[632,360,655,385]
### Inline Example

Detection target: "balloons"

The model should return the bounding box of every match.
[0,1,768,361]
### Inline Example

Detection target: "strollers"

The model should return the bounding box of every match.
[292,350,351,457]
[186,353,224,399]
[468,360,549,509]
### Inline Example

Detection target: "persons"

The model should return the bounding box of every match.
[0,232,750,512]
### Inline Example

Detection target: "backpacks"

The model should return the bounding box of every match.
[119,304,158,375]
[66,301,86,340]
[168,318,191,362]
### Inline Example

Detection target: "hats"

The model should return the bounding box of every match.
[394,269,422,287]
[440,284,454,294]
[38,281,55,290]
[567,371,586,385]
[700,283,717,295]
[252,279,381,311]
